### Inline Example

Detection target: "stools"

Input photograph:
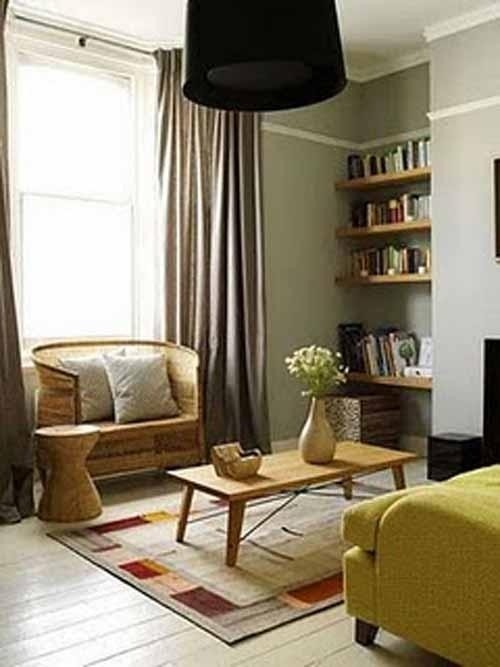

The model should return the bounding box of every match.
[33,426,104,520]
[324,394,403,449]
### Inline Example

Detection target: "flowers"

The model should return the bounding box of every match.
[284,344,348,401]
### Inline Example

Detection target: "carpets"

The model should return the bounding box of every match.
[47,476,401,643]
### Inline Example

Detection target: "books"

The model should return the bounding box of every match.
[349,243,430,278]
[352,192,431,228]
[348,138,430,180]
[337,321,432,378]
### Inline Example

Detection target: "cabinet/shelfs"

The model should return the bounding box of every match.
[336,165,435,283]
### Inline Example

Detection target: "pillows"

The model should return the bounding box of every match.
[59,345,181,420]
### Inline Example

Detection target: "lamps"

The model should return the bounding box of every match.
[182,0,351,110]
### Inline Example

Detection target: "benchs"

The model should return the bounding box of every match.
[29,338,207,484]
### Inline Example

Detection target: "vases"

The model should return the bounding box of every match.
[300,394,335,464]
